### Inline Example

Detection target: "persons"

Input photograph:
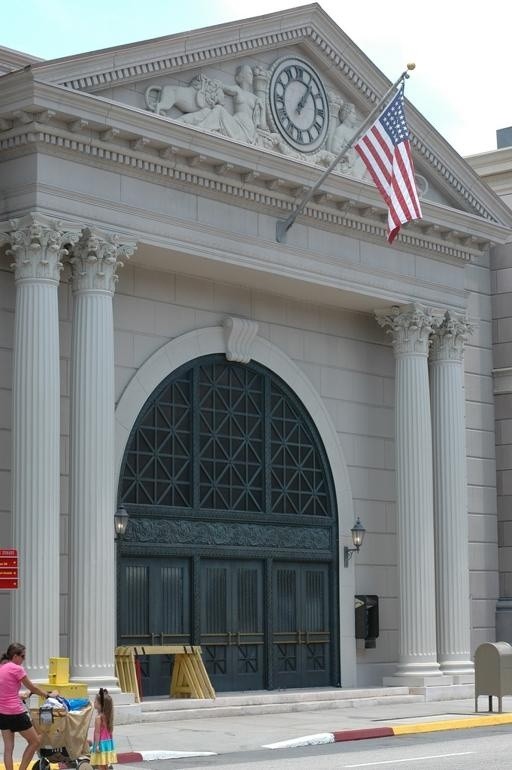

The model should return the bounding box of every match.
[89,688,118,770]
[0,643,60,770]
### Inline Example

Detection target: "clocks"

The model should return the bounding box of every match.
[265,56,332,154]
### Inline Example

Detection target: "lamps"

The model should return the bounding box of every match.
[344,517,366,568]
[115,502,129,540]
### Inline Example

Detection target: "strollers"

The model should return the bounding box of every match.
[23,691,94,770]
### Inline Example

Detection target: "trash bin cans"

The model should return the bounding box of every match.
[475,641,512,713]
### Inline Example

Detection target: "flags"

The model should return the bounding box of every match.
[352,83,422,245]
